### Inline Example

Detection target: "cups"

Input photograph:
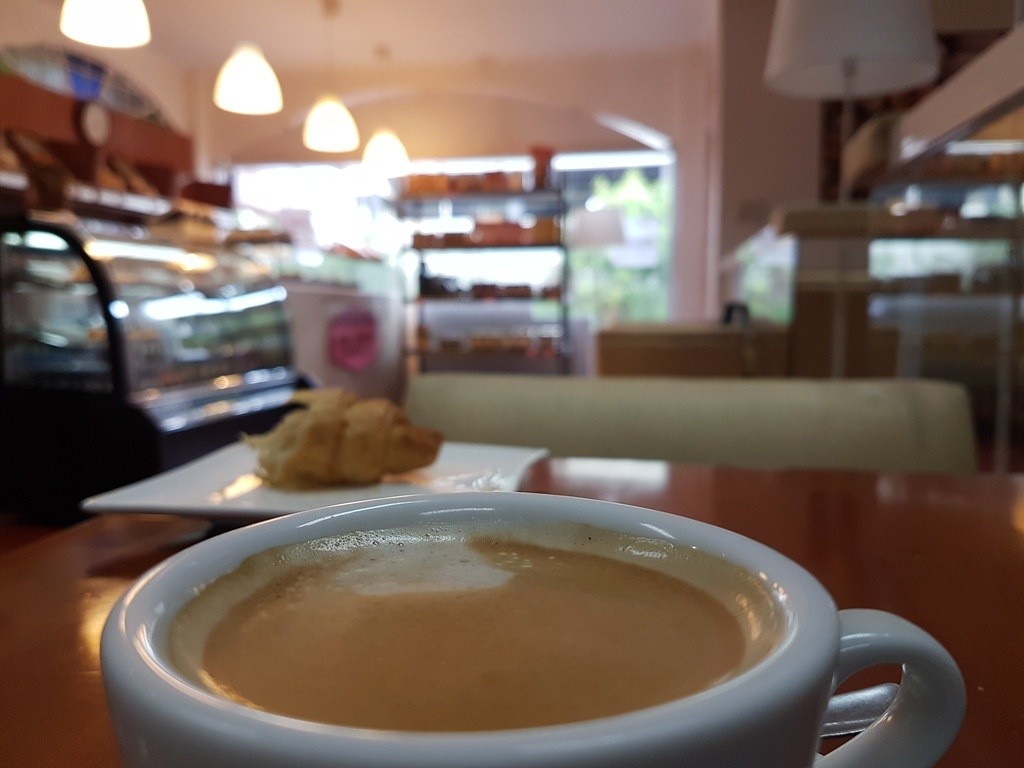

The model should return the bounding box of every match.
[100,489,965,767]
[288,267,407,412]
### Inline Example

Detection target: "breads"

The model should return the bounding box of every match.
[247,387,443,486]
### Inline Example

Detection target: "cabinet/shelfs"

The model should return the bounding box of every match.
[403,191,568,350]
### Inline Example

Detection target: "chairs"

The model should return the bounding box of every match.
[410,377,976,467]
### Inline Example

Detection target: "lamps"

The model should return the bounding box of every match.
[761,0,936,375]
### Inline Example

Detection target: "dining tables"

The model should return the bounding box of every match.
[0,463,1024,768]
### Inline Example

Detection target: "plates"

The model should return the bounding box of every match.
[82,433,551,516]
